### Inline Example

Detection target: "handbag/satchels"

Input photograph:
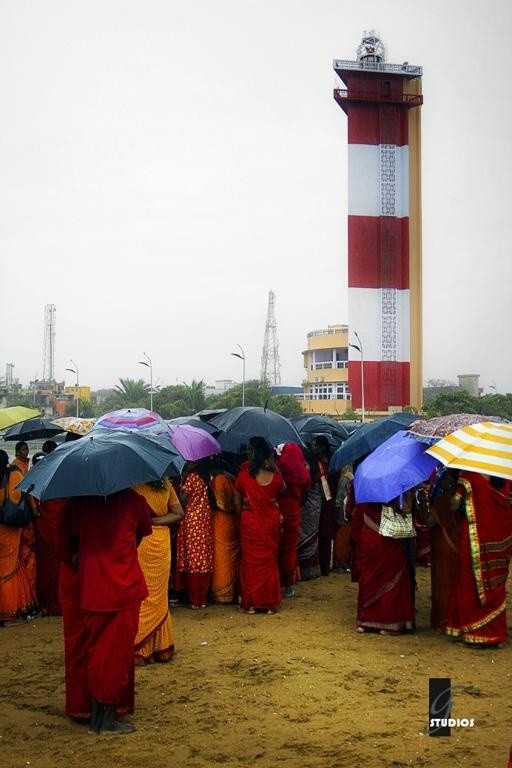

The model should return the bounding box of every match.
[316,459,333,502]
[0,464,37,529]
[378,503,418,540]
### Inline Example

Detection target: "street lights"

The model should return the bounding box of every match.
[30,378,40,409]
[488,380,499,416]
[347,330,366,424]
[139,350,154,412]
[65,359,80,419]
[230,343,246,408]
